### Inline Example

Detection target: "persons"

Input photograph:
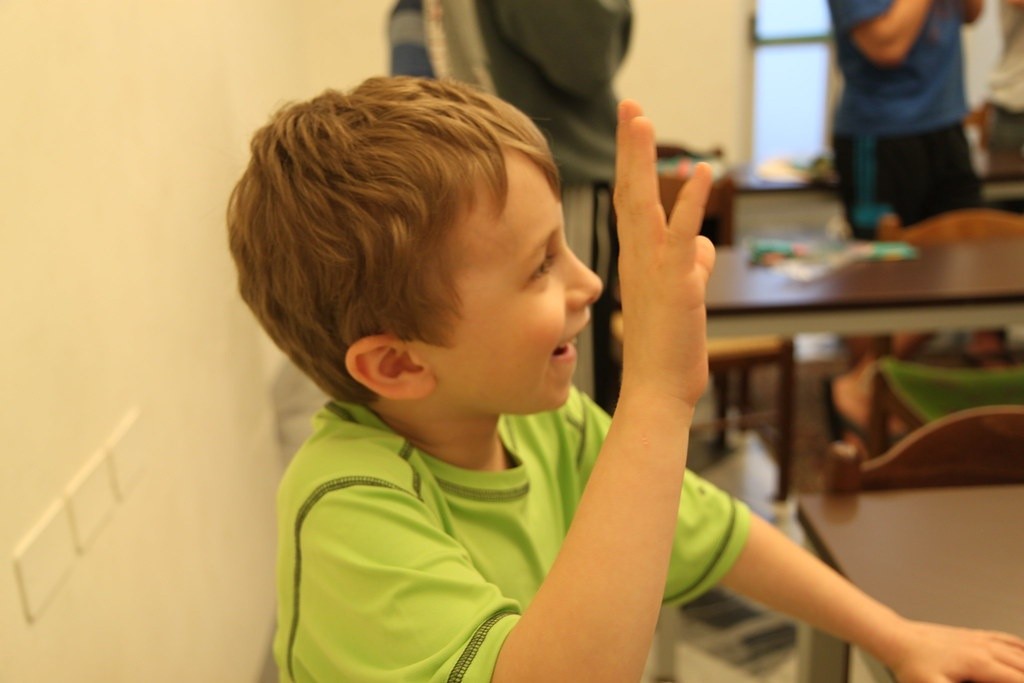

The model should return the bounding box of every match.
[478,2,638,403]
[828,1,1005,419]
[224,73,1022,681]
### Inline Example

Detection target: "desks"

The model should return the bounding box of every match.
[785,489,1024,683]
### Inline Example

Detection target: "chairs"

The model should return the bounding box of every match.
[585,136,795,504]
[700,206,1024,486]
